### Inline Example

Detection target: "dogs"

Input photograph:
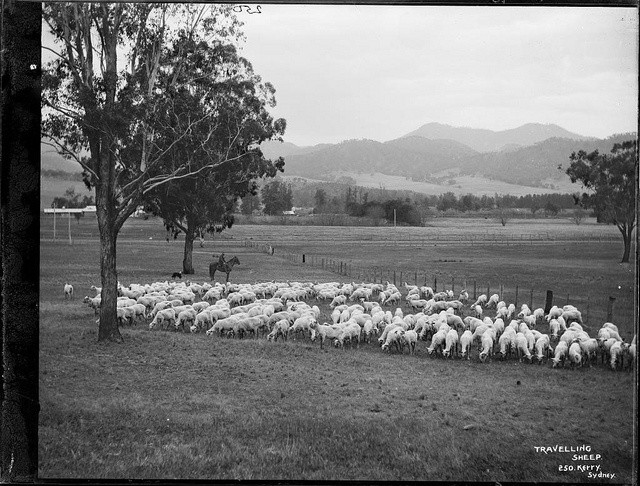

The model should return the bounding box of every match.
[171,272,182,280]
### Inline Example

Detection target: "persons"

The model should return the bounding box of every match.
[219,253,229,274]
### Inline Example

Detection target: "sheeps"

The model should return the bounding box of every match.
[83,280,636,372]
[63,282,74,301]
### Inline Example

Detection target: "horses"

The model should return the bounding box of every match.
[209,256,241,282]
